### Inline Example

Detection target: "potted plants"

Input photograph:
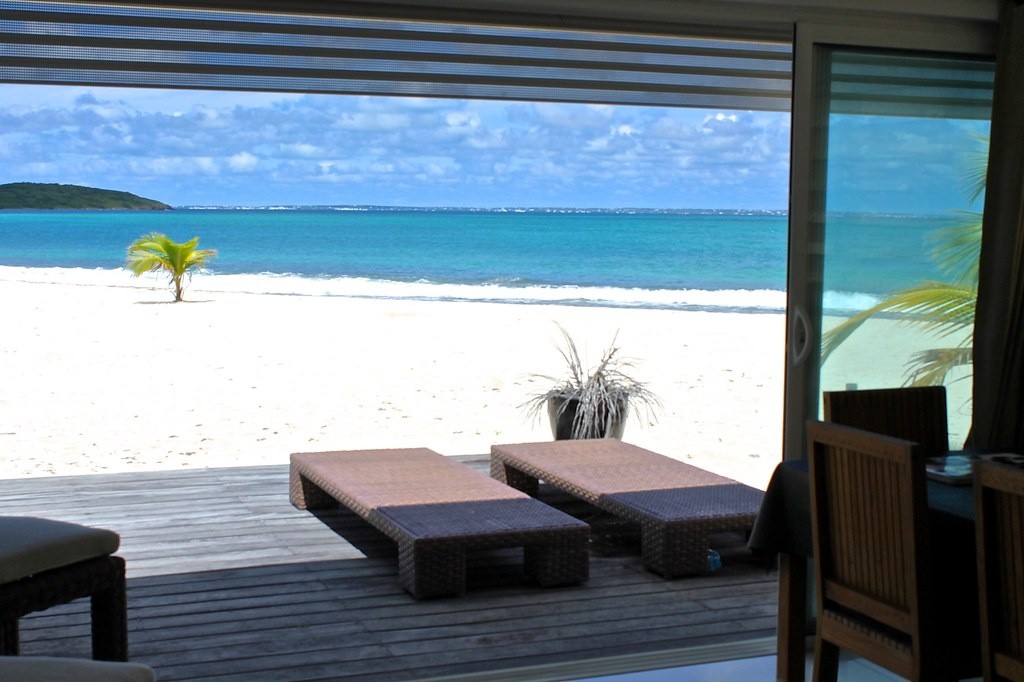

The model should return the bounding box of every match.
[520,320,664,439]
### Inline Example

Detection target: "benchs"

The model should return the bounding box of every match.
[291,447,591,600]
[491,438,767,582]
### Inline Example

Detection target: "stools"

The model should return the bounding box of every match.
[0,516,126,663]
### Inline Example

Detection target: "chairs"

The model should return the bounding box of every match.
[805,386,1024,682]
